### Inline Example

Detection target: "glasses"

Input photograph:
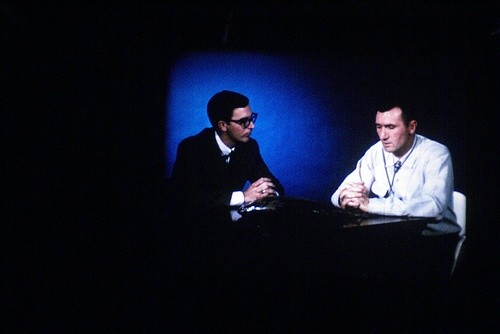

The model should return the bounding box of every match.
[221,112,259,129]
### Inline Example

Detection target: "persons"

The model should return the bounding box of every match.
[330,98,462,242]
[172,90,286,209]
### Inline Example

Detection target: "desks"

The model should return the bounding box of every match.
[236,196,429,262]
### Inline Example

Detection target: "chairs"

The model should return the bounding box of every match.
[435,191,467,281]
